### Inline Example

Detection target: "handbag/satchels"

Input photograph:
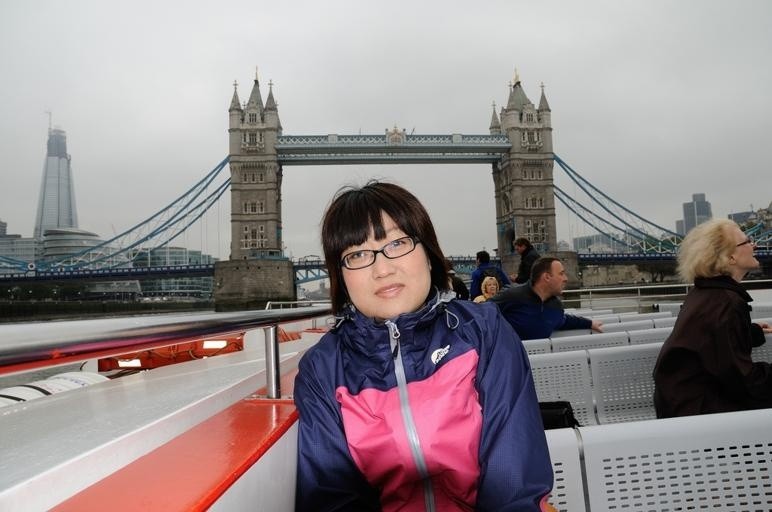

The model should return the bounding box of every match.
[539,402,580,430]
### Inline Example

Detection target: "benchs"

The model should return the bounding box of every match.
[515,305,772,512]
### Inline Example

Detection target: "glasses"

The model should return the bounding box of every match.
[736,235,755,247]
[340,235,422,270]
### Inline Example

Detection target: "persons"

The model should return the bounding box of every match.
[631,274,637,293]
[650,217,772,476]
[293,177,561,512]
[478,255,605,341]
[470,250,512,301]
[445,259,469,300]
[473,276,499,304]
[641,277,646,293]
[510,237,541,285]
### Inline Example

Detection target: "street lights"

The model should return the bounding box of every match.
[7,289,211,303]
